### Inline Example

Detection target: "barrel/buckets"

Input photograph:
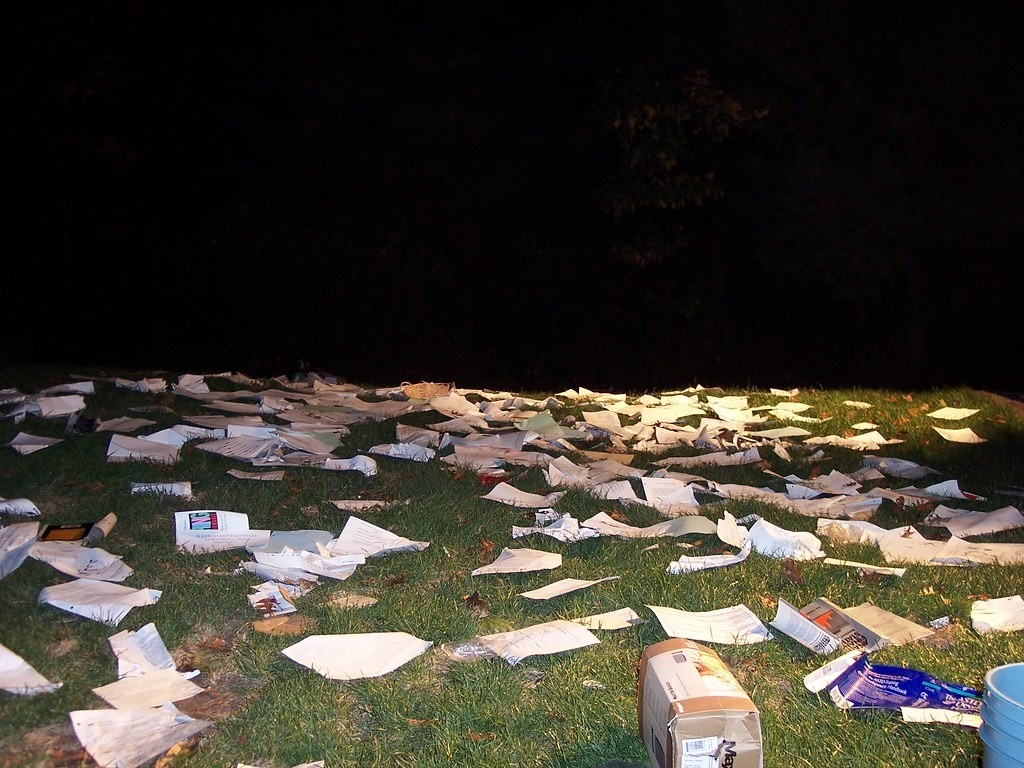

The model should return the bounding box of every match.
[978,663,1024,768]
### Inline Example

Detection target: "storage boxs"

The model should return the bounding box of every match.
[637,637,766,768]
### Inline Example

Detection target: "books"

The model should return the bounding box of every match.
[35,511,118,548]
[771,597,935,660]
